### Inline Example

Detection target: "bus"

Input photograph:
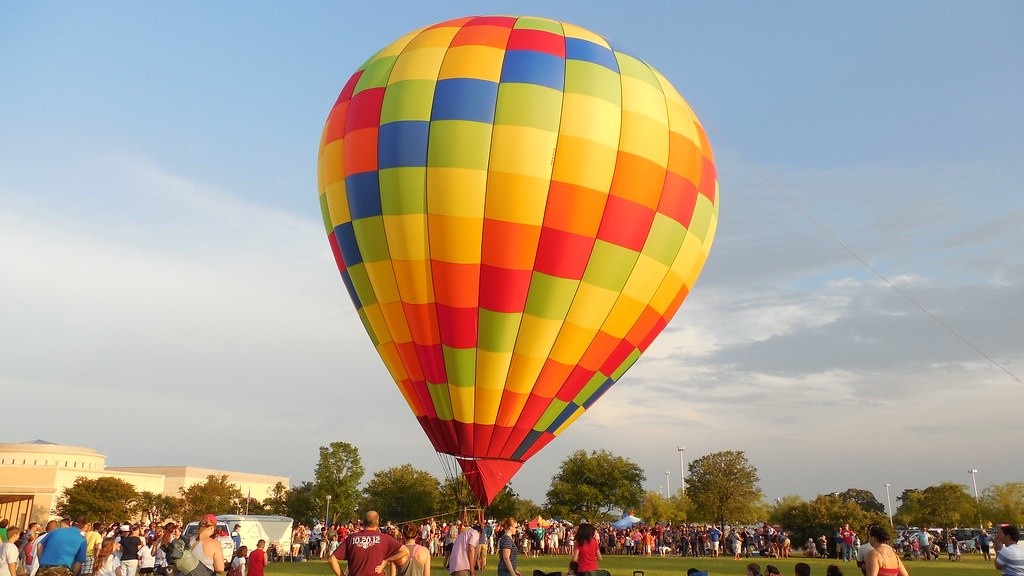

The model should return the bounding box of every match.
[890,525,1024,554]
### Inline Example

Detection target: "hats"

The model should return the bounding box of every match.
[202,514,217,525]
[165,523,179,529]
[120,525,130,531]
[456,520,461,525]
[235,524,241,527]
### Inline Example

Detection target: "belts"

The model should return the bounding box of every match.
[39,564,68,568]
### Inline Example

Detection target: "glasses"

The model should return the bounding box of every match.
[513,526,518,529]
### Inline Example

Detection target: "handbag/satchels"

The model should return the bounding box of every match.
[732,534,744,542]
[166,538,215,576]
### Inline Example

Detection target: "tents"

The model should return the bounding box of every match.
[611,514,641,529]
[529,515,574,530]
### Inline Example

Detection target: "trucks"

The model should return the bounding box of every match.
[180,513,294,563]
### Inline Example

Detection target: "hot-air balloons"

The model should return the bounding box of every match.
[316,14,720,530]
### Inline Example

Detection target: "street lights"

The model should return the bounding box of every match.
[885,483,893,528]
[677,444,685,496]
[665,471,670,499]
[325,495,332,526]
[968,469,984,530]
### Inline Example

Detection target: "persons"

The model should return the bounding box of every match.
[994,526,1024,576]
[866,527,909,576]
[497,517,522,576]
[449,525,483,576]
[328,510,410,576]
[390,522,431,576]
[764,565,783,576]
[0,514,277,576]
[572,523,602,576]
[687,568,708,576]
[827,565,844,576]
[566,561,578,576]
[290,517,792,570]
[856,523,881,576]
[747,563,763,576]
[795,563,810,576]
[803,522,1005,563]
